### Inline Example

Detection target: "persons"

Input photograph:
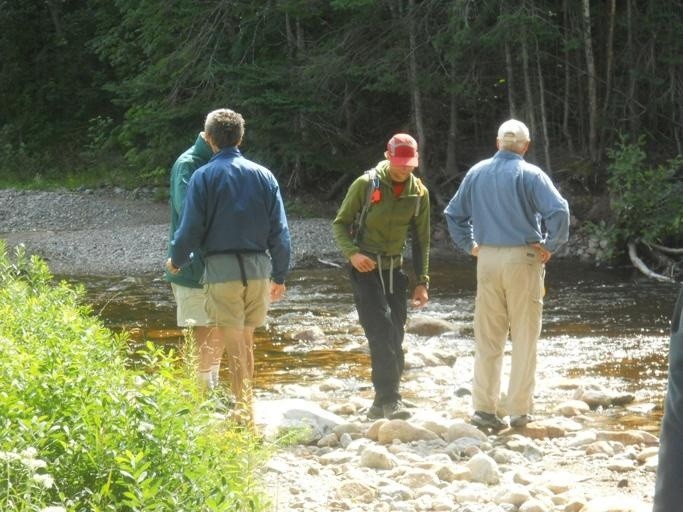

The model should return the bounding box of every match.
[165,131,236,414]
[164,108,291,437]
[329,131,433,419]
[440,118,571,431]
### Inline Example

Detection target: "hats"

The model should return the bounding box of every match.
[388,133,419,168]
[498,119,531,143]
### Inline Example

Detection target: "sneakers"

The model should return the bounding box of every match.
[470,409,507,429]
[366,399,414,418]
[510,414,532,425]
[214,386,237,408]
[193,391,230,413]
[383,400,413,421]
